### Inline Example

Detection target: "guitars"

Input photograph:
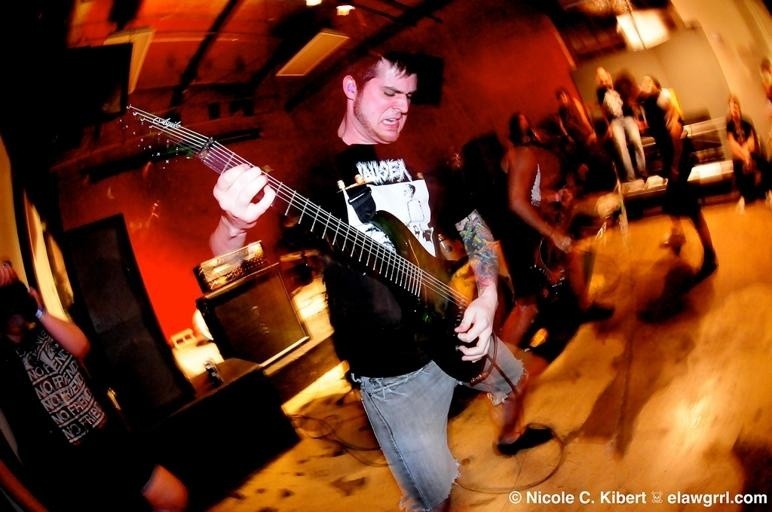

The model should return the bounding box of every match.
[120,103,515,382]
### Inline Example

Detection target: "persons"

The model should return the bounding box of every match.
[203,38,554,512]
[1,256,190,511]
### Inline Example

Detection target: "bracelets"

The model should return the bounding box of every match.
[35,302,45,319]
[220,215,248,238]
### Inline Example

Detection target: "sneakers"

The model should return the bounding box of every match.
[495,427,553,457]
[701,249,719,272]
[579,302,615,323]
